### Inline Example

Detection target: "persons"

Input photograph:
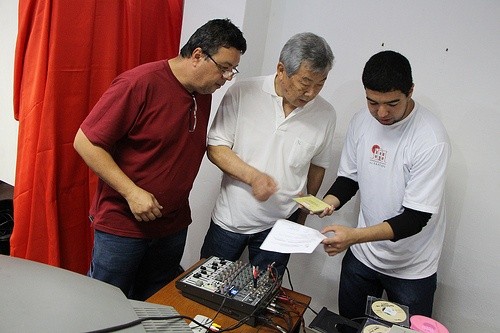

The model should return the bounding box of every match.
[298,50,449,327]
[200,32,337,286]
[72,18,247,304]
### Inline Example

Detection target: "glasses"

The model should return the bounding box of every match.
[202,51,239,80]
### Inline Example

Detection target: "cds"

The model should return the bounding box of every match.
[362,301,450,333]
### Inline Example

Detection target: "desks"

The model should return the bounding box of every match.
[146,259,313,333]
